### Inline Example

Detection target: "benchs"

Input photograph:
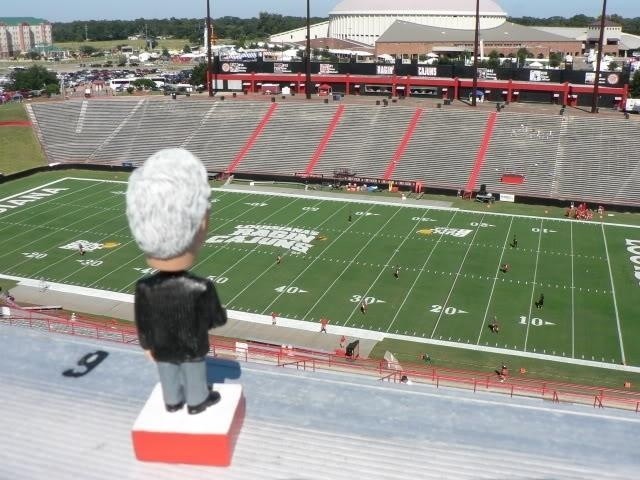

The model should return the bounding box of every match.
[25,96,640,208]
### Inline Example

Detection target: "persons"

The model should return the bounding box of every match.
[319,318,330,333]
[360,301,368,314]
[394,264,399,280]
[499,365,507,383]
[339,335,346,350]
[124,147,228,415]
[401,375,412,386]
[79,243,85,255]
[491,202,605,331]
[71,313,77,321]
[277,255,281,263]
[270,311,277,325]
